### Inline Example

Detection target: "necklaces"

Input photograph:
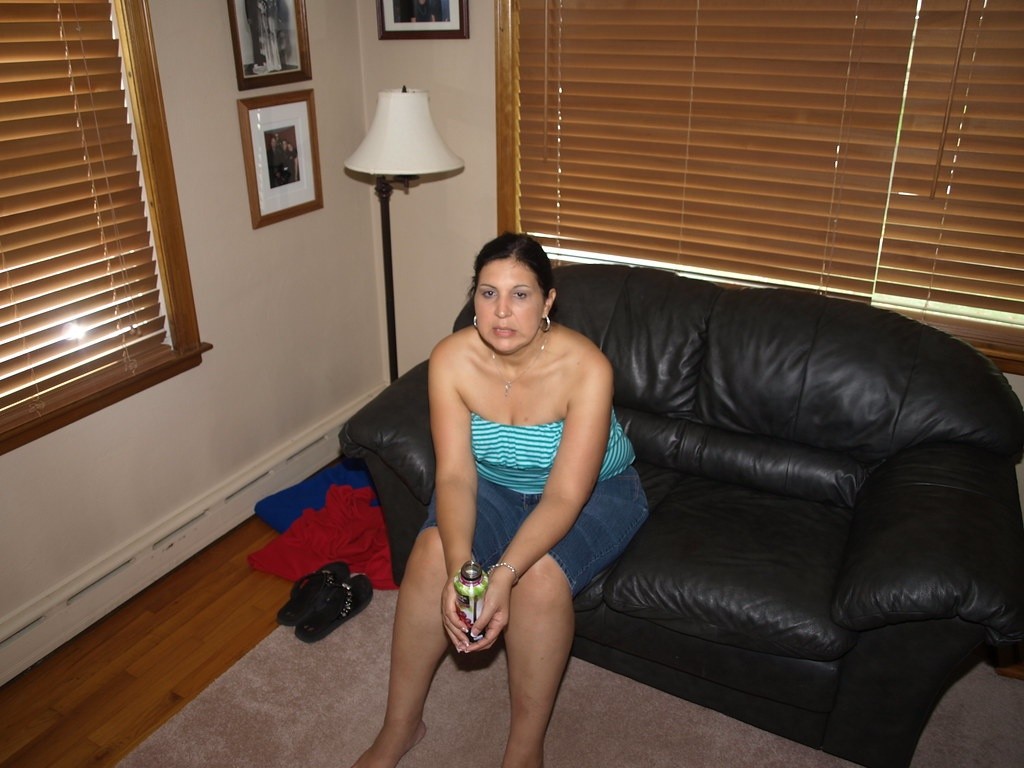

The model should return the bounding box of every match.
[491,324,552,398]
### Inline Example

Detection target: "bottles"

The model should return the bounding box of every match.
[453,561,489,642]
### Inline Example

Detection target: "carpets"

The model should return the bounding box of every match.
[113,587,1024,768]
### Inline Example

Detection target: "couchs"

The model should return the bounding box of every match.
[336,264,1024,768]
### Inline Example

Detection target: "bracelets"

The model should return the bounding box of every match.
[485,563,519,586]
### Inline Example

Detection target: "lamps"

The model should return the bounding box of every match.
[346,85,465,384]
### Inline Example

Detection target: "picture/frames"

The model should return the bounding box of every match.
[236,88,324,231]
[376,0,470,40]
[227,0,312,91]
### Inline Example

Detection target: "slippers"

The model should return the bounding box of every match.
[277,561,373,643]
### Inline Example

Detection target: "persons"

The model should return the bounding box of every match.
[268,131,297,187]
[410,0,441,22]
[352,230,651,768]
